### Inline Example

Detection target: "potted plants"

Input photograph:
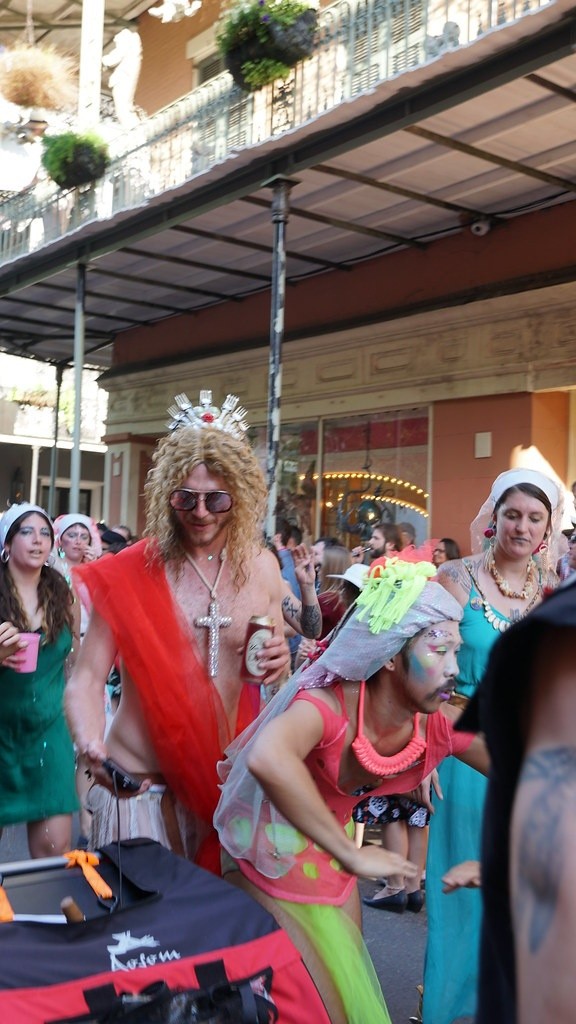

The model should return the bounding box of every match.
[42,133,107,190]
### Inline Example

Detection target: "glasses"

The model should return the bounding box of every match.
[168,485,235,514]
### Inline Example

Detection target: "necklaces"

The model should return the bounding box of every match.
[351,681,427,776]
[463,559,540,622]
[183,545,233,678]
[486,548,538,600]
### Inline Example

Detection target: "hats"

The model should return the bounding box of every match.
[0,501,54,549]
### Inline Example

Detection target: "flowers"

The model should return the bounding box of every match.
[216,0,317,91]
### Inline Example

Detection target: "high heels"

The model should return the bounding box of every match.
[408,887,425,914]
[363,888,408,912]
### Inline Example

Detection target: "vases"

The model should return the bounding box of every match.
[223,9,317,92]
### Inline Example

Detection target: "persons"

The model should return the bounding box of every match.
[62,391,294,883]
[421,468,562,1024]
[0,503,82,862]
[259,517,459,909]
[45,513,146,714]
[449,573,576,1024]
[102,28,142,130]
[207,553,490,1024]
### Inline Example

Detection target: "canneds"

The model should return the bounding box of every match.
[238,616,276,683]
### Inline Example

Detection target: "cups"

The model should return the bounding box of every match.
[15,632,41,672]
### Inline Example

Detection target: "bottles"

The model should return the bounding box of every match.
[241,615,275,684]
